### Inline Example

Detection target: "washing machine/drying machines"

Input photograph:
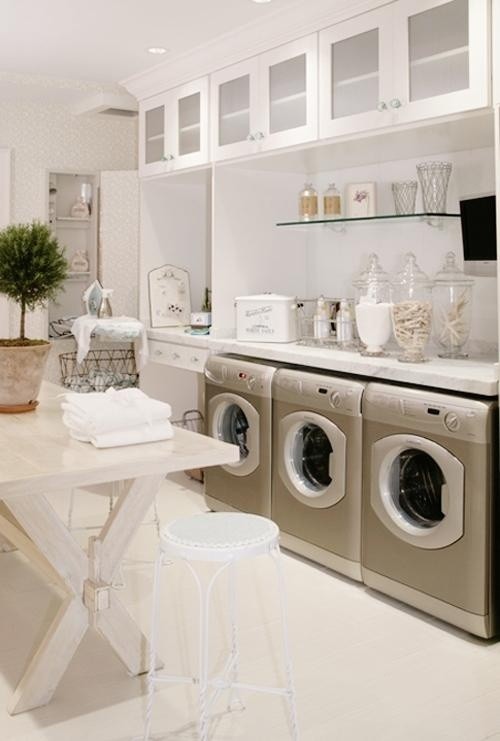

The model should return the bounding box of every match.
[360,381,500,641]
[272,365,369,582]
[204,353,285,517]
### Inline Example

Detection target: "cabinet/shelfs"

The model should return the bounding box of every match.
[208,35,319,161]
[320,2,489,141]
[49,215,95,338]
[137,75,206,177]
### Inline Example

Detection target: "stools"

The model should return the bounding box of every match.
[145,511,293,735]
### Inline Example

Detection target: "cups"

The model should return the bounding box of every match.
[390,179,418,214]
[415,161,451,213]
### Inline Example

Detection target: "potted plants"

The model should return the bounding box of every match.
[0,224,67,413]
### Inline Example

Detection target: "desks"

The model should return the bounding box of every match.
[0,379,241,717]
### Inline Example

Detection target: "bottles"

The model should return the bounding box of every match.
[323,181,342,220]
[70,249,89,271]
[70,194,88,218]
[312,293,332,339]
[334,297,354,341]
[297,181,317,222]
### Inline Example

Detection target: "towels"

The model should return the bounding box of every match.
[62,387,173,448]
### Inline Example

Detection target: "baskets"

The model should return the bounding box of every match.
[59,349,139,392]
